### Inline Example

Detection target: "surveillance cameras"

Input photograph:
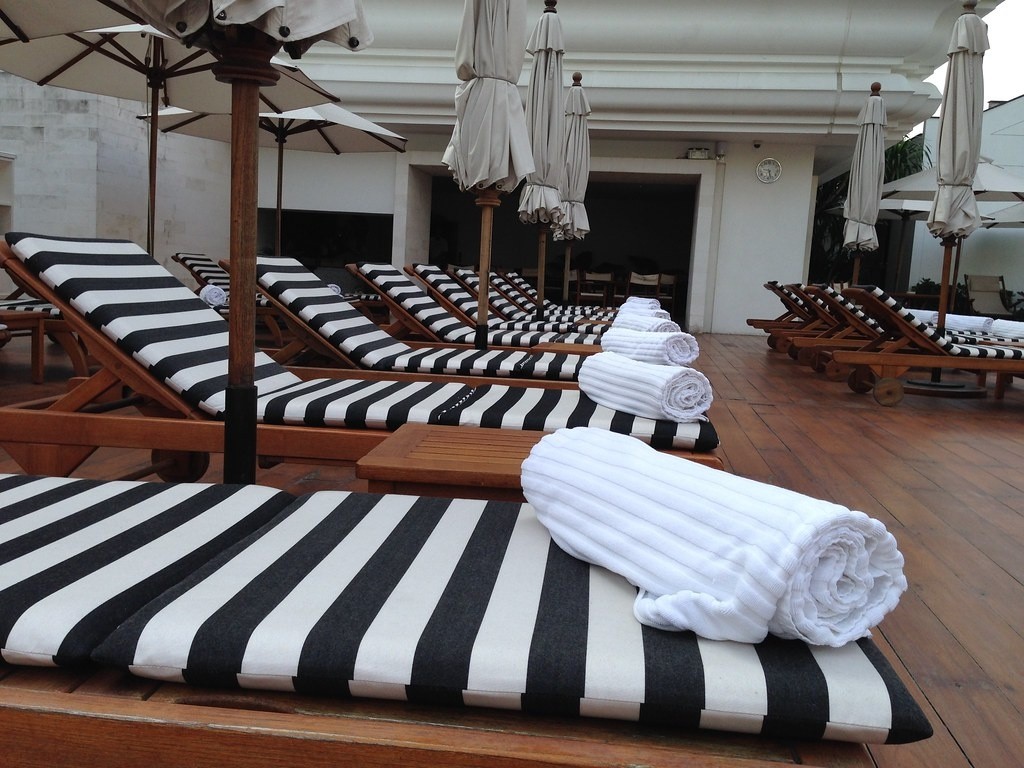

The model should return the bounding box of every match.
[754,140,761,148]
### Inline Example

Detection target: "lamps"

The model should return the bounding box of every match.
[716,141,726,160]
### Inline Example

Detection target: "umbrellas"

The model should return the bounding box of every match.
[125,0,379,488]
[550,73,592,303]
[1,24,342,261]
[135,102,408,258]
[515,0,567,322]
[882,155,1024,314]
[978,202,1024,230]
[442,0,536,350]
[840,79,888,287]
[0,0,146,46]
[925,1,990,383]
[827,198,995,297]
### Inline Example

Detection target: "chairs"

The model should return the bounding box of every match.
[0,231,1024,768]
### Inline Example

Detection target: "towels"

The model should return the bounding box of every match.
[520,426,908,649]
[991,319,1024,339]
[619,302,661,310]
[578,351,713,423]
[327,284,341,294]
[601,327,700,367]
[611,313,682,333]
[944,313,994,333]
[625,296,661,307]
[904,309,938,324]
[200,285,227,307]
[616,308,672,322]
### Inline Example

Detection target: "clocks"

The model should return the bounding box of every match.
[755,158,782,183]
